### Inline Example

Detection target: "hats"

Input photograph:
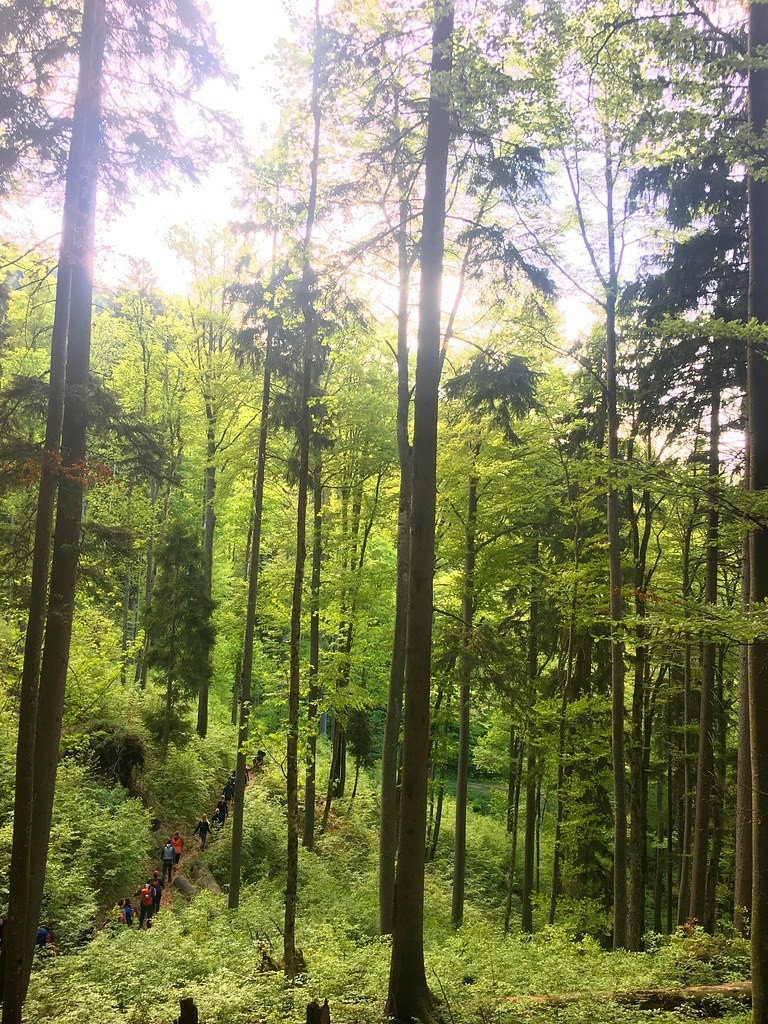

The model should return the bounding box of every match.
[154,870,158,874]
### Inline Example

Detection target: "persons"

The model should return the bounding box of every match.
[35,762,250,947]
[171,831,185,872]
[193,813,212,852]
[161,839,175,884]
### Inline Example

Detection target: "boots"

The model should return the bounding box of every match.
[162,874,172,883]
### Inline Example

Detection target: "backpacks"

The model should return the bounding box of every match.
[47,931,55,944]
[141,888,152,904]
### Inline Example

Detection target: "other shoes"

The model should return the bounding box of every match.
[173,865,180,872]
